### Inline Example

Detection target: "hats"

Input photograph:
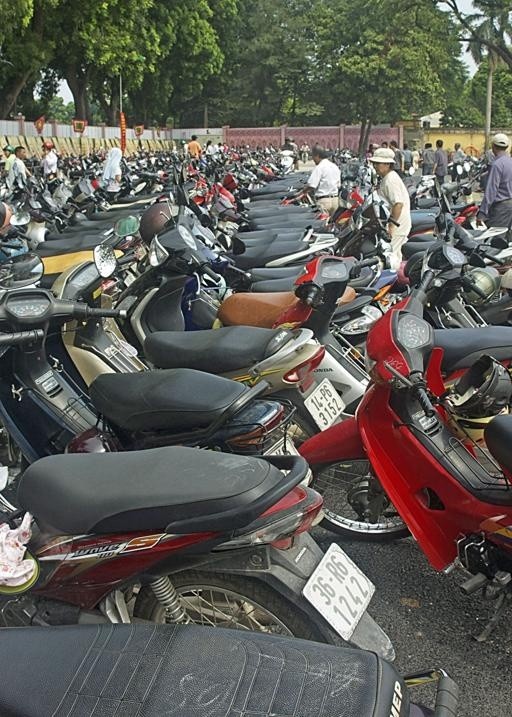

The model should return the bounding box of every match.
[369,148,396,163]
[490,133,507,147]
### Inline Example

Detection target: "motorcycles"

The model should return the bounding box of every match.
[299,269,512,644]
[0,626,456,717]
[0,138,512,437]
[0,444,397,668]
[43,243,347,451]
[0,287,314,485]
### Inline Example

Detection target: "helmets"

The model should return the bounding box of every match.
[44,142,53,149]
[450,355,512,418]
[0,513,40,596]
[65,427,118,452]
[462,265,501,307]
[139,201,175,244]
[4,145,14,153]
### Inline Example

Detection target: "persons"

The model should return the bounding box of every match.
[180,135,228,169]
[0,201,11,228]
[369,148,412,269]
[367,141,466,182]
[281,138,309,162]
[104,147,122,199]
[12,146,31,188]
[0,147,16,189]
[476,134,512,241]
[303,145,342,218]
[40,142,57,178]
